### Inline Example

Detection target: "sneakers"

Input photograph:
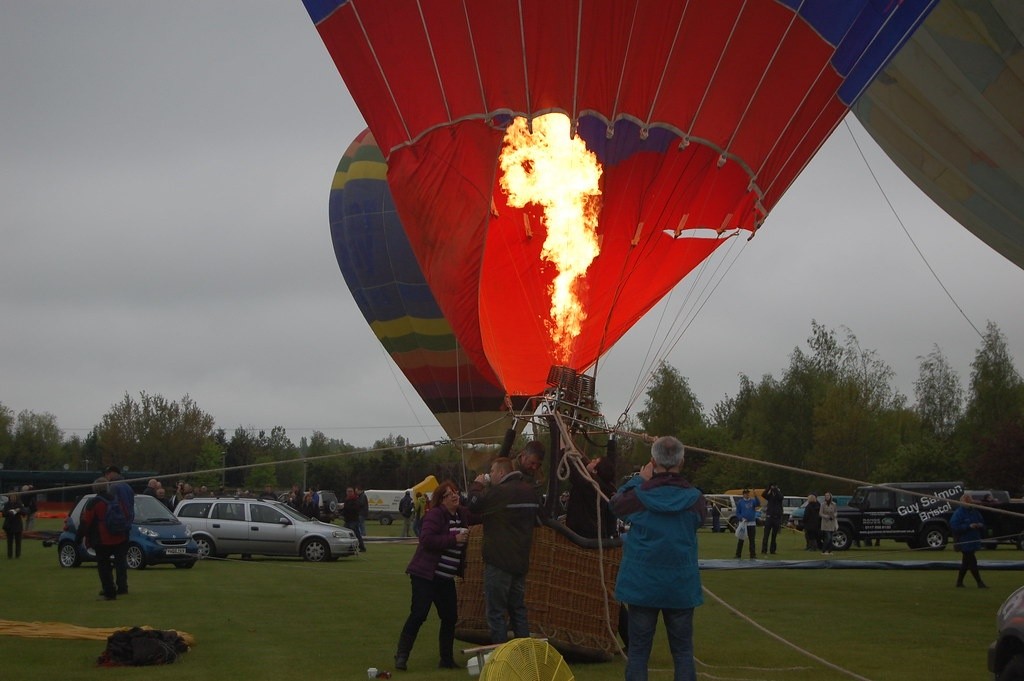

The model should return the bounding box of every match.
[438,658,462,671]
[395,653,409,672]
[99,587,128,601]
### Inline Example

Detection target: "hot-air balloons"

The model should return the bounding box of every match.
[296,1,1024,660]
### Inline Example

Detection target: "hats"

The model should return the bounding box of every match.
[824,489,833,493]
[809,492,817,497]
[742,487,750,494]
[102,466,121,473]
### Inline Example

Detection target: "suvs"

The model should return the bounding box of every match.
[279,491,340,523]
[818,482,963,552]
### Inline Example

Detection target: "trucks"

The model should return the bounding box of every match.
[363,490,435,525]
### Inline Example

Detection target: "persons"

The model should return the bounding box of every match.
[762,483,783,554]
[951,494,988,588]
[800,491,880,554]
[395,441,618,671]
[0,466,367,601]
[733,490,761,558]
[607,437,707,681]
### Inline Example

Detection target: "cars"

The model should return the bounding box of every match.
[985,584,1024,681]
[56,492,198,570]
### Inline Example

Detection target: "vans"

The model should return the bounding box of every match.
[700,494,745,533]
[174,496,360,562]
[761,497,811,525]
[788,495,866,530]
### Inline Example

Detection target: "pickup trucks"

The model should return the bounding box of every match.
[963,490,1024,550]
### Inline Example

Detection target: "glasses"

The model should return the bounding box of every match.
[442,490,456,496]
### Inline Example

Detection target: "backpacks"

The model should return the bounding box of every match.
[104,501,133,537]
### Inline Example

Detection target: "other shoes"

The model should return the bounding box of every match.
[735,555,741,559]
[771,551,777,554]
[760,551,768,555]
[750,556,757,559]
[977,584,989,588]
[806,546,834,554]
[956,582,964,588]
